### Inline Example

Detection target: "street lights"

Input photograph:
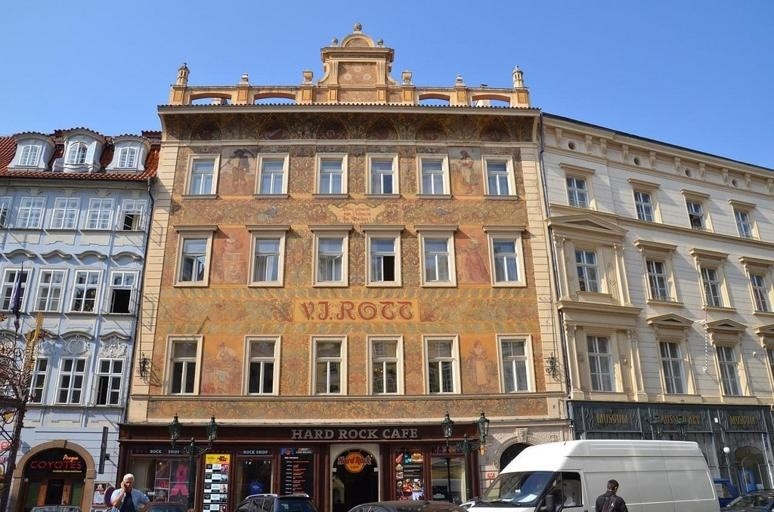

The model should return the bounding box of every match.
[169,412,217,511]
[442,410,489,502]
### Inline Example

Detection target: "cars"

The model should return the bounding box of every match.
[30,506,82,512]
[720,490,774,512]
[149,502,187,512]
[347,501,469,512]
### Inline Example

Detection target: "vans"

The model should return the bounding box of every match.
[713,478,739,508]
[454,439,721,512]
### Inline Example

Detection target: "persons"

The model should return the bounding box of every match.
[110,474,150,511]
[596,478,628,512]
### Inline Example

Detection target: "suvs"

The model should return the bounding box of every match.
[234,492,319,512]
